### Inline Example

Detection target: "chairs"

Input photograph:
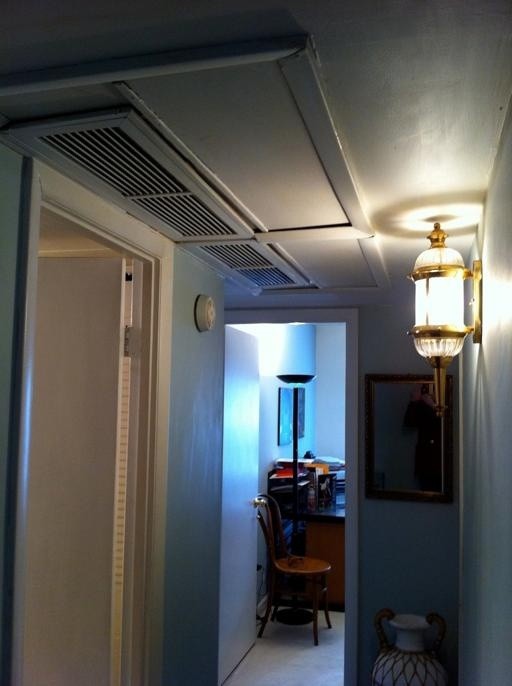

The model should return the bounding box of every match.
[256,493,332,646]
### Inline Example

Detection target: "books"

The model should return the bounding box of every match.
[269,456,345,514]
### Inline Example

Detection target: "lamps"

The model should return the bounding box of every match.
[412,222,486,415]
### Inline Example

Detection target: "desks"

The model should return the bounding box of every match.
[280,492,345,609]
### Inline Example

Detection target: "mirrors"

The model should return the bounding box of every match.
[363,374,454,504]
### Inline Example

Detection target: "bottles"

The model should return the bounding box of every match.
[306,485,315,511]
